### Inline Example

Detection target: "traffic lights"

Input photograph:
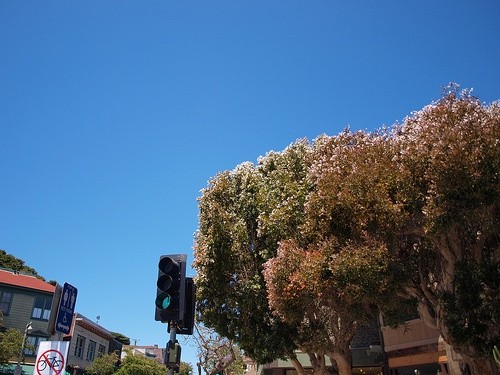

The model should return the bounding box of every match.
[155,254,188,321]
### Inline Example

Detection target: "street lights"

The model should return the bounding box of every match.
[16,322,33,375]
